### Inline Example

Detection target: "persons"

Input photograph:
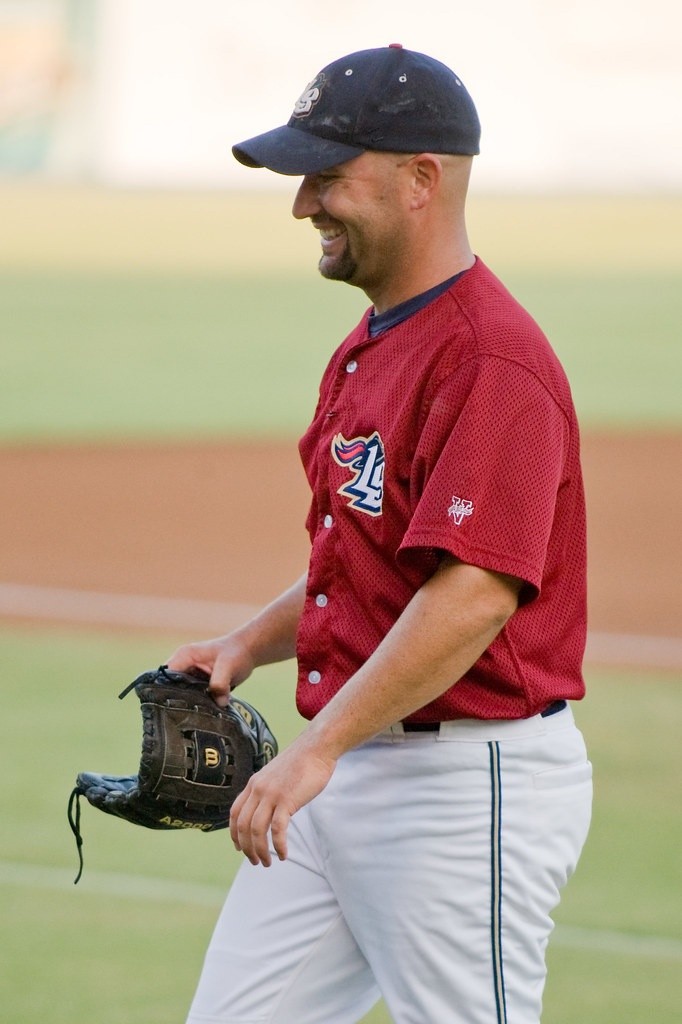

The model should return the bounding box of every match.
[64,44,593,1024]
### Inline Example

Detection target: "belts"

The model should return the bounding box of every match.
[402,698,567,733]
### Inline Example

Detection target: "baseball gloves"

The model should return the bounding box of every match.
[76,669,278,832]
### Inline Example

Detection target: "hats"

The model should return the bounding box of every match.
[232,44,480,175]
